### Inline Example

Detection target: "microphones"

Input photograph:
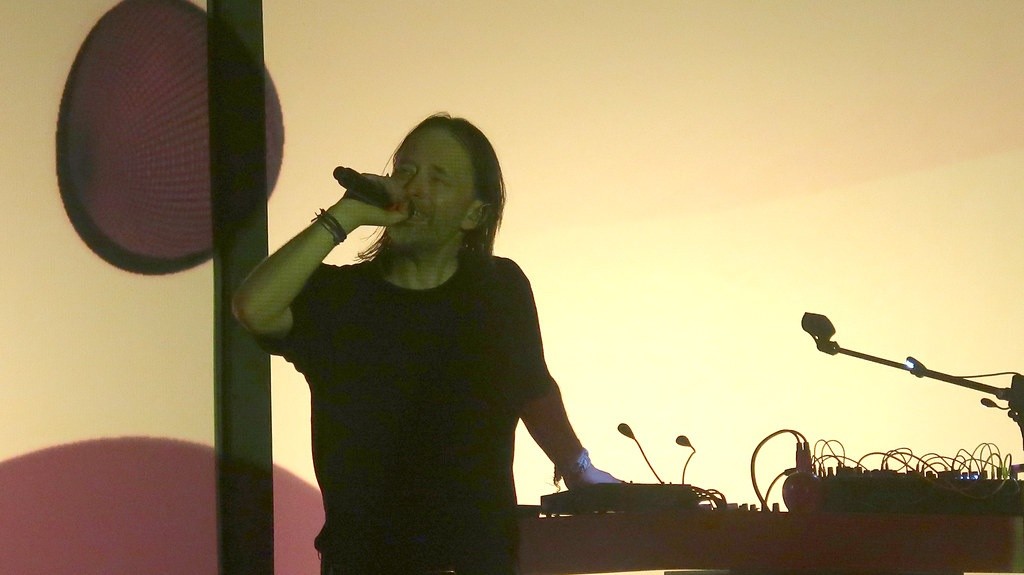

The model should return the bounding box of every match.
[333,166,414,219]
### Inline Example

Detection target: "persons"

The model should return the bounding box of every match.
[231,115,625,575]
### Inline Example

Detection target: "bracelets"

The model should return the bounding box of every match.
[318,210,347,243]
[554,447,589,483]
